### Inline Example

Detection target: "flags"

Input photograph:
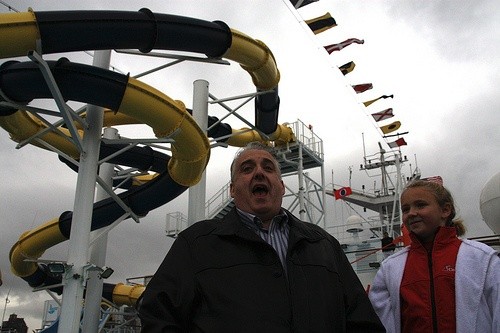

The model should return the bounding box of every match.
[387,138,407,148]
[352,83,373,94]
[363,94,393,107]
[305,12,338,35]
[339,61,355,76]
[371,108,394,122]
[334,187,352,200]
[289,0,319,10]
[323,38,364,55]
[380,121,401,134]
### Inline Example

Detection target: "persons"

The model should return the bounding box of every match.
[135,142,387,333]
[368,180,500,333]
